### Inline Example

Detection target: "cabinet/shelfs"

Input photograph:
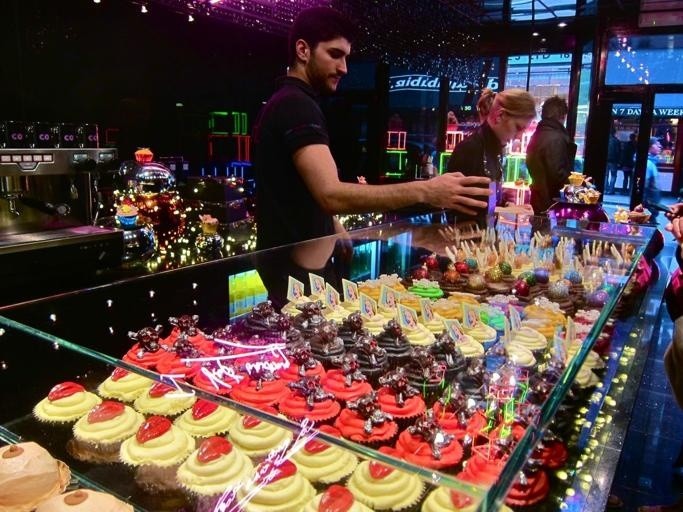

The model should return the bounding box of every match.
[0,208,680,512]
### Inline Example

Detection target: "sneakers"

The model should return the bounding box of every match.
[604,189,633,195]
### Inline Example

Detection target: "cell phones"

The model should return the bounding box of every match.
[647,199,674,214]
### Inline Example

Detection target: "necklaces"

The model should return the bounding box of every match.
[478,135,502,179]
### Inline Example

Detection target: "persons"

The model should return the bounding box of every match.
[603,128,622,195]
[632,137,662,224]
[447,88,536,217]
[257,236,353,312]
[254,5,496,250]
[432,111,461,146]
[525,96,577,220]
[663,199,683,469]
[388,112,402,142]
[621,133,637,193]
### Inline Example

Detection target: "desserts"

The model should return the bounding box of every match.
[0,204,663,512]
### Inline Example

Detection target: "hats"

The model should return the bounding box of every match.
[649,136,664,147]
